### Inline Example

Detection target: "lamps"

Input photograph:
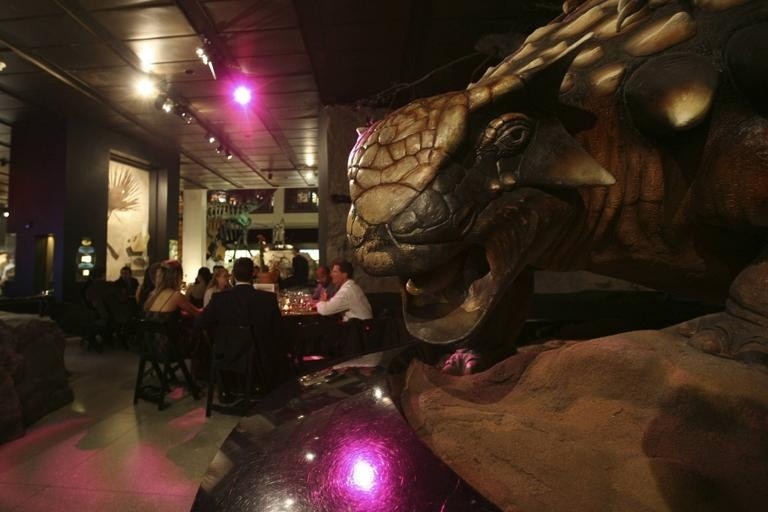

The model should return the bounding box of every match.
[78,238,97,276]
[154,38,234,160]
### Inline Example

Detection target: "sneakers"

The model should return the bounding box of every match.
[220,392,235,403]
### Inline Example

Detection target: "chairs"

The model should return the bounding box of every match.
[327,310,386,364]
[134,316,201,413]
[203,319,285,417]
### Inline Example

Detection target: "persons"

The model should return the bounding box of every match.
[312,265,340,356]
[310,256,381,361]
[86,257,294,404]
[292,247,310,286]
